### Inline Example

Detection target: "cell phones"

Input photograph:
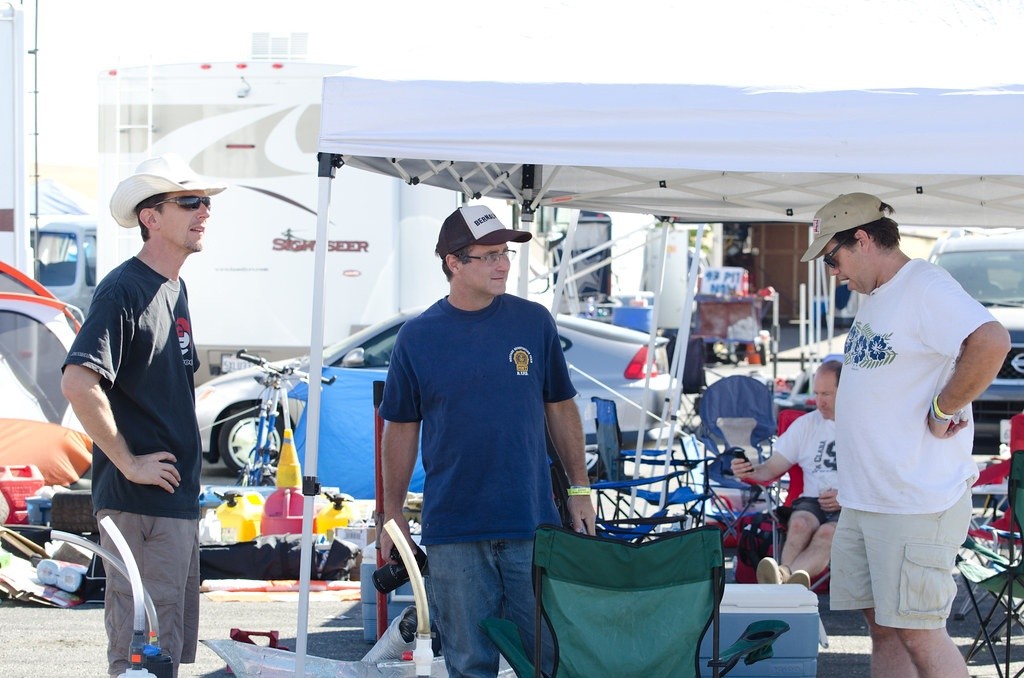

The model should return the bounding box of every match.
[733,449,755,472]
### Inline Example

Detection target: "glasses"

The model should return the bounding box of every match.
[458,250,517,265]
[824,230,871,269]
[154,196,210,210]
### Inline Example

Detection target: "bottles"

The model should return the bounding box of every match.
[276,428,302,489]
[316,492,355,535]
[215,490,256,542]
[260,488,304,535]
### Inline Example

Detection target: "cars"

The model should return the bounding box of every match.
[194,300,681,475]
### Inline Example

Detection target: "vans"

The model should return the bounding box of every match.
[548,209,723,333]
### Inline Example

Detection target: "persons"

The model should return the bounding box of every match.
[731,361,841,588]
[58,158,227,678]
[376,205,595,678]
[800,193,1010,678]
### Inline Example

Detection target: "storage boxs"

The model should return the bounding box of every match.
[25,496,50,526]
[698,582,821,678]
[336,525,414,640]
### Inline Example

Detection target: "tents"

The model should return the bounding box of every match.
[296,77,1023,659]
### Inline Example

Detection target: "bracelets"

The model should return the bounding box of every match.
[567,485,591,496]
[930,401,950,424]
[933,393,953,419]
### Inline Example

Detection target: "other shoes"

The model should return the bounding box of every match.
[786,570,811,591]
[756,555,782,584]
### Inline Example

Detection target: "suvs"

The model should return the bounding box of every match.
[924,230,1024,441]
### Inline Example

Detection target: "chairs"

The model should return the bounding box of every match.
[477,376,1024,678]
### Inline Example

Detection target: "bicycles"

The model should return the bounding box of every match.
[236,348,337,485]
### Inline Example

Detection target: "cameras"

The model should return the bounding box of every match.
[371,539,428,595]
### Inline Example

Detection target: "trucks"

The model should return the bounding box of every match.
[30,170,565,386]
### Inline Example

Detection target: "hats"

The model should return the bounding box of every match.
[440,205,532,258]
[800,192,885,262]
[110,158,227,228]
